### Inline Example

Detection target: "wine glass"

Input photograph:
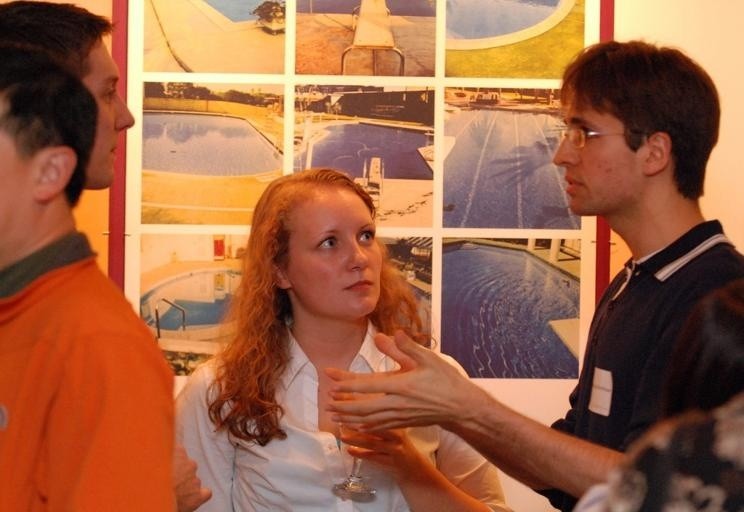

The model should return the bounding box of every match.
[330,393,390,497]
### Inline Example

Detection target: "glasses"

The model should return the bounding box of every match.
[561,129,649,148]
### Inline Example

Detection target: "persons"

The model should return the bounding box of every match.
[0,1,214,510]
[324,39,742,512]
[173,171,506,511]
[571,283,740,512]
[1,47,174,511]
